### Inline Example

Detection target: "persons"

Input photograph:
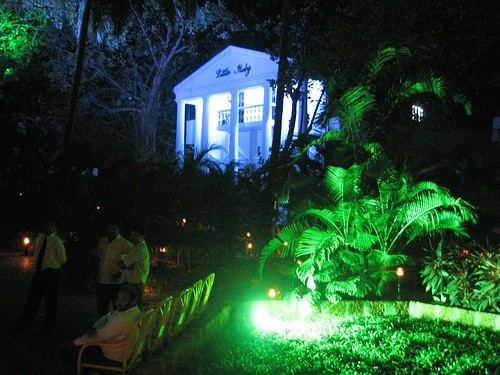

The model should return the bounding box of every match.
[15,215,69,337]
[118,226,150,304]
[87,219,136,316]
[63,284,145,374]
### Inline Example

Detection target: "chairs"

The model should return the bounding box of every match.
[201,272,216,311]
[170,286,195,337]
[146,295,173,359]
[193,279,203,317]
[77,309,155,375]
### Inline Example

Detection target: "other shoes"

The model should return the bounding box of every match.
[40,325,57,334]
[13,322,28,332]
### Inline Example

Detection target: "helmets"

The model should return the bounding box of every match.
[117,283,138,298]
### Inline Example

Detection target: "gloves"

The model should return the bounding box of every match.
[73,336,87,346]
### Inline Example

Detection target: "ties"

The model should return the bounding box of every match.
[37,237,47,272]
